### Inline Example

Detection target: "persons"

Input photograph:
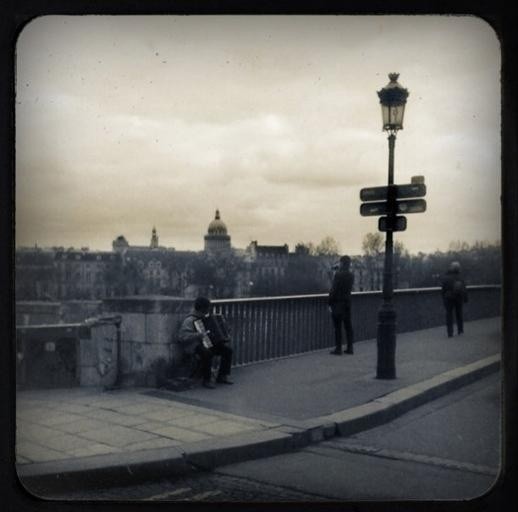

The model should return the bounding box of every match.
[326,255,355,355]
[177,297,234,388]
[440,260,469,337]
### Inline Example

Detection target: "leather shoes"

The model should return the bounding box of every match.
[216,375,234,383]
[344,350,353,354]
[202,379,217,388]
[331,351,341,354]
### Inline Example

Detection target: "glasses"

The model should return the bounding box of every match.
[201,311,208,314]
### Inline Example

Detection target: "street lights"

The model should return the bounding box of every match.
[359,74,426,378]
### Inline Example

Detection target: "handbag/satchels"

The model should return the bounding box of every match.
[453,280,466,298]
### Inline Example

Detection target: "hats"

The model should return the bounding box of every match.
[448,262,461,273]
